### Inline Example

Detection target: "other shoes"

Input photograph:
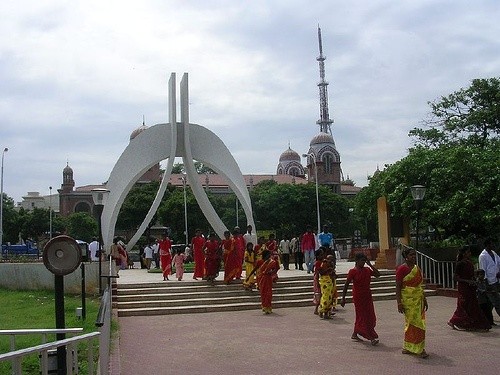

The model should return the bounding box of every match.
[402,351,412,354]
[351,336,363,342]
[314,310,336,320]
[371,340,379,345]
[416,349,429,358]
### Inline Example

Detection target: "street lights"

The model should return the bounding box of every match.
[302,154,321,234]
[0,147,8,254]
[178,177,189,247]
[48,186,53,240]
[410,185,427,250]
[349,207,355,238]
[90,183,110,293]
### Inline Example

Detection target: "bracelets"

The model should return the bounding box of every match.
[397,302,402,305]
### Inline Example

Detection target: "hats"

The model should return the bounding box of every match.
[234,226,240,229]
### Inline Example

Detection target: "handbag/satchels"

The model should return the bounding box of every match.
[96,250,100,257]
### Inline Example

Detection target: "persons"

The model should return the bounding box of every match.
[245,250,280,313]
[342,252,381,345]
[83,225,278,291]
[473,270,493,332]
[447,246,477,332]
[395,249,430,358]
[477,237,500,319]
[280,224,338,320]
